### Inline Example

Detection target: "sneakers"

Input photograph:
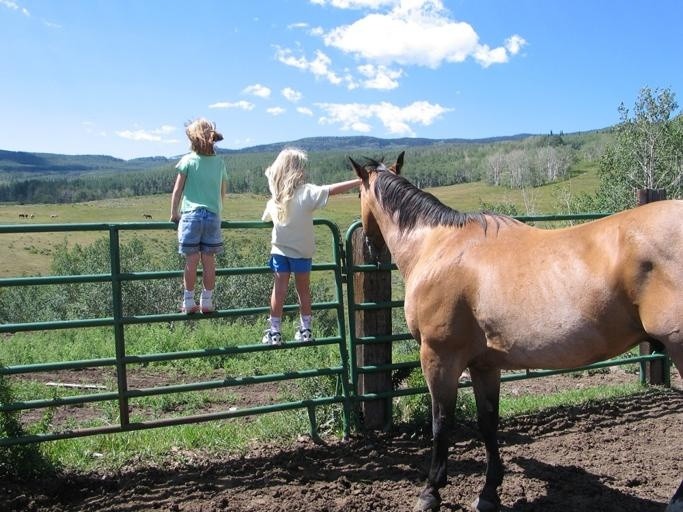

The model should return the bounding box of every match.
[200,299,215,313]
[294,327,313,343]
[262,331,282,347]
[182,298,199,314]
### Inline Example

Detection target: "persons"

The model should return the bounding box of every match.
[259,145,361,348]
[166,119,230,317]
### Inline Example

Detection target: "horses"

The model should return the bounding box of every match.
[142,213,152,220]
[19,213,58,219]
[344,149,682,512]
